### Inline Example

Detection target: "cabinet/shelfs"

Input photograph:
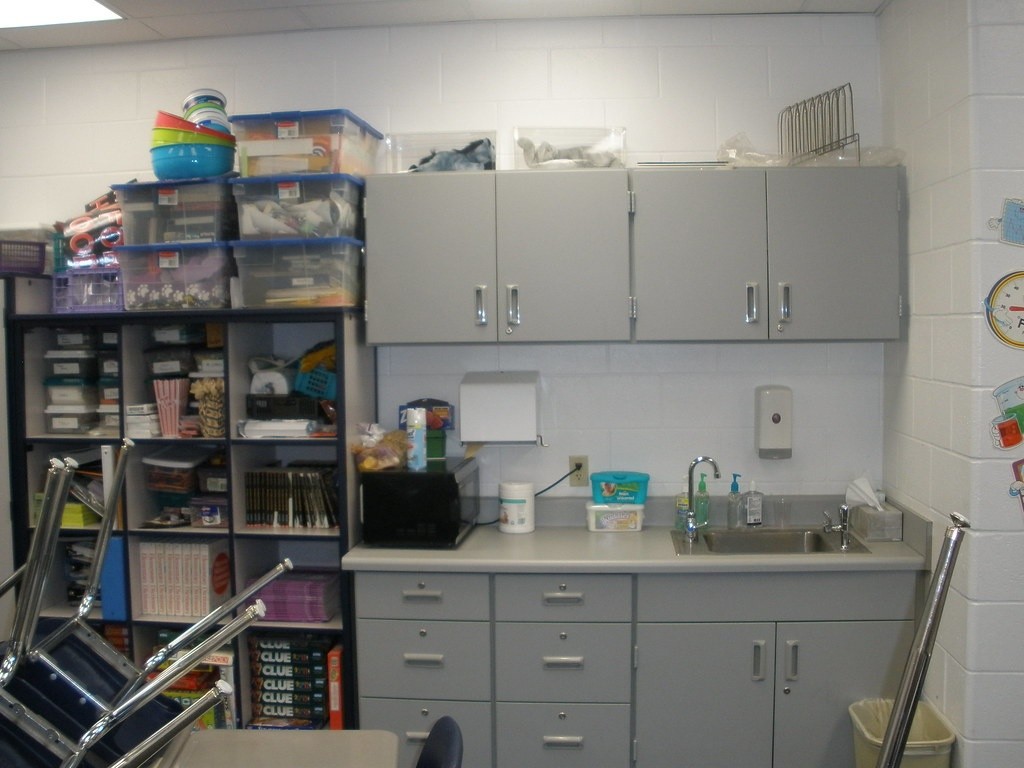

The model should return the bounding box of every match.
[354,572,927,768]
[364,169,633,345]
[630,166,902,341]
[3,275,360,729]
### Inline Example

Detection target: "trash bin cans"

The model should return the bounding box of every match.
[848,698,956,768]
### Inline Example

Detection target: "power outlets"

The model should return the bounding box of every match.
[569,454,589,485]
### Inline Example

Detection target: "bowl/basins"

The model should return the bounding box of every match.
[150,88,236,179]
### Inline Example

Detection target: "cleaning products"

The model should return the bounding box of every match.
[695,472,710,529]
[675,475,689,529]
[727,472,764,530]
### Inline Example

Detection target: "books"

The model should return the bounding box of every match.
[139,538,232,622]
[247,569,340,623]
[244,460,347,528]
[250,636,354,731]
[152,639,236,666]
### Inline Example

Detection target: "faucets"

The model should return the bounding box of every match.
[686,456,722,541]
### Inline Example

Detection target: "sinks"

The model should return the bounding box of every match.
[702,529,837,554]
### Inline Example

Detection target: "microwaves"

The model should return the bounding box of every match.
[360,455,480,549]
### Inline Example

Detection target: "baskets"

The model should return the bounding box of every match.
[52,233,124,310]
[0,238,48,276]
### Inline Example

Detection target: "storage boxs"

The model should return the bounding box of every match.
[108,180,239,246]
[248,391,323,419]
[51,233,125,314]
[41,325,121,435]
[227,177,365,240]
[228,236,365,308]
[126,324,225,438]
[137,440,230,529]
[111,240,237,312]
[228,109,385,175]
[0,239,50,275]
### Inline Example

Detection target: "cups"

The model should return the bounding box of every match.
[498,480,535,534]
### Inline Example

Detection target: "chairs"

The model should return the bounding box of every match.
[415,716,463,768]
[0,437,293,768]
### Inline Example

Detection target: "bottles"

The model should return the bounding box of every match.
[405,407,427,469]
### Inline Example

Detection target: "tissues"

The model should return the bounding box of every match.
[845,478,903,542]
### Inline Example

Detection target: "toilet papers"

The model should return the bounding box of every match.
[249,369,296,395]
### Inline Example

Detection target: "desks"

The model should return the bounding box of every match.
[157,729,400,768]
